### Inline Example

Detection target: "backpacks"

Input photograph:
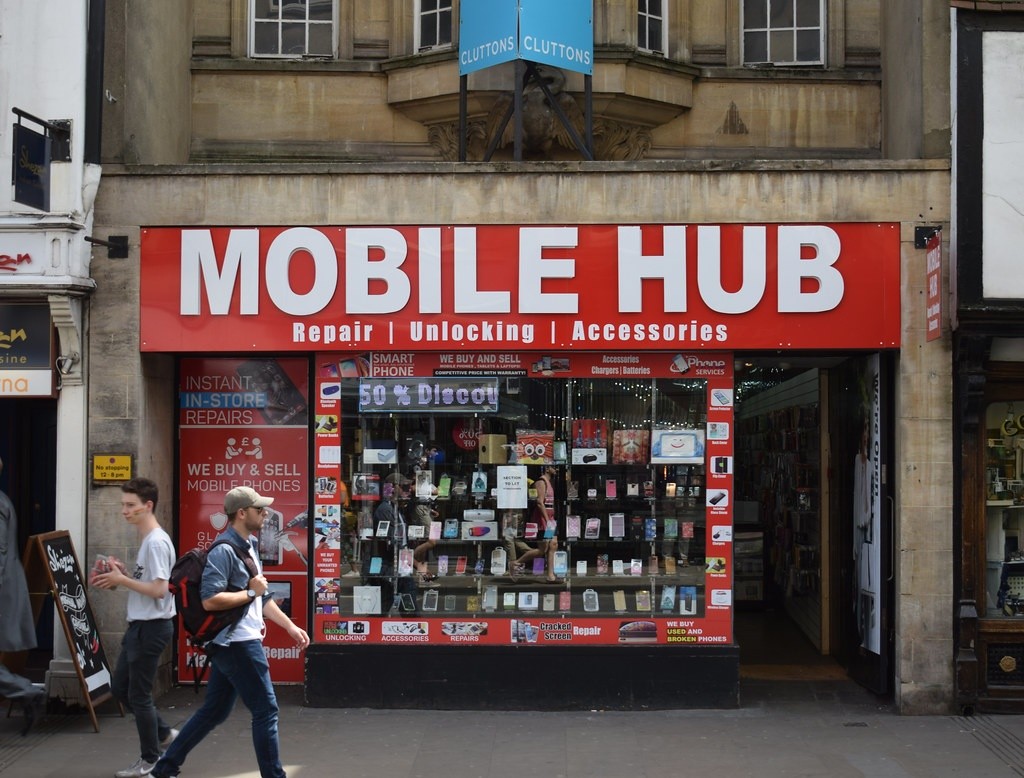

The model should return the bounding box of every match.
[523,478,547,519]
[357,510,393,580]
[169,539,259,645]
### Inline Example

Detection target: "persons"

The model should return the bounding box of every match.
[710,424,718,437]
[361,472,422,617]
[501,509,534,576]
[145,485,311,778]
[509,464,565,583]
[663,464,693,568]
[91,478,180,778]
[338,482,361,579]
[410,441,440,562]
[0,459,51,738]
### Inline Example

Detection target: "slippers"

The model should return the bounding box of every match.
[508,562,517,582]
[547,576,566,583]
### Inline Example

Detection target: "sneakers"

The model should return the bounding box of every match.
[115,755,162,778]
[158,728,179,758]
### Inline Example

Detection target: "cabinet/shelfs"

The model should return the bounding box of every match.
[341,460,707,618]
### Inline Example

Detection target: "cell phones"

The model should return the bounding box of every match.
[715,457,728,473]
[322,505,327,517]
[674,354,690,374]
[321,353,371,379]
[353,622,364,633]
[685,593,692,612]
[531,356,552,372]
[713,391,729,405]
[709,493,726,505]
[257,509,279,567]
[328,506,333,516]
[510,618,539,643]
[235,358,307,426]
[618,621,658,643]
[320,480,335,494]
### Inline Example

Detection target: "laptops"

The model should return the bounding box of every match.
[378,451,394,461]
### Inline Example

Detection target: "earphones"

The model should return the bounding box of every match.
[406,626,410,628]
[403,622,408,625]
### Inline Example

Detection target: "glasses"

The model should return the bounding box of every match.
[398,486,410,492]
[549,465,557,470]
[255,507,263,514]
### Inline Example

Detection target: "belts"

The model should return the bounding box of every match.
[416,502,430,505]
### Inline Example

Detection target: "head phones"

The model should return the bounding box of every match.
[408,440,423,459]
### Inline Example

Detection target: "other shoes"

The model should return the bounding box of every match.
[502,569,512,576]
[659,556,669,567]
[681,559,689,566]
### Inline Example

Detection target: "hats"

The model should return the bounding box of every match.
[385,472,414,485]
[224,486,274,514]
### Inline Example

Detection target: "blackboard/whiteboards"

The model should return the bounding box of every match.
[0,529,114,707]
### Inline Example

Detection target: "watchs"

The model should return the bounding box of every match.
[244,587,256,602]
